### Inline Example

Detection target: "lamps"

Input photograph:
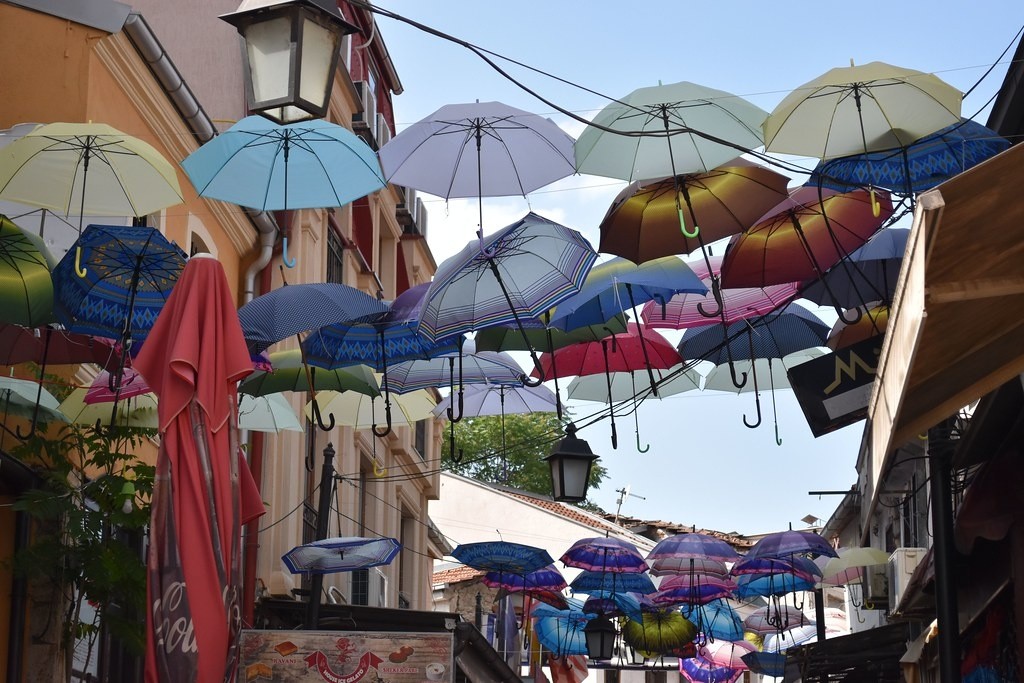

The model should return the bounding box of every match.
[577,608,621,662]
[216,0,365,126]
[541,419,603,503]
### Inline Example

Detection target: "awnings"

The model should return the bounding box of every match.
[887,459,1024,623]
[856,141,1024,548]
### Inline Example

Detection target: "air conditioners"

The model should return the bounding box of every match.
[395,186,417,228]
[377,112,392,149]
[886,547,927,616]
[860,563,889,611]
[412,196,428,238]
[352,79,378,142]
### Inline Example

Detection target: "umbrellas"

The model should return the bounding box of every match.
[0,60,1011,452]
[281,474,400,575]
[131,251,267,683]
[449,522,892,683]
